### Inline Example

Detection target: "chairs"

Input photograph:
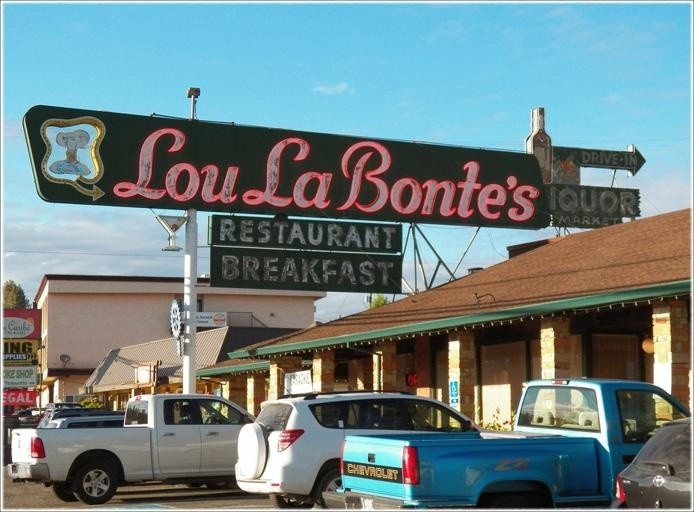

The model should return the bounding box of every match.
[176,404,193,425]
[577,411,598,427]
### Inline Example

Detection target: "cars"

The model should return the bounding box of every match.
[4,401,126,466]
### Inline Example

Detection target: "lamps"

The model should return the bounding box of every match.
[641,331,656,354]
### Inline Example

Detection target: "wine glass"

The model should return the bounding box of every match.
[156,214,187,251]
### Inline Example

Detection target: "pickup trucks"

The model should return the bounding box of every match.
[319,374,691,510]
[7,392,262,507]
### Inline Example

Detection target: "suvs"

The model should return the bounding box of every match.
[609,417,692,510]
[232,390,490,510]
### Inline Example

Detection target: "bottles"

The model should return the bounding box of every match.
[528,108,553,183]
